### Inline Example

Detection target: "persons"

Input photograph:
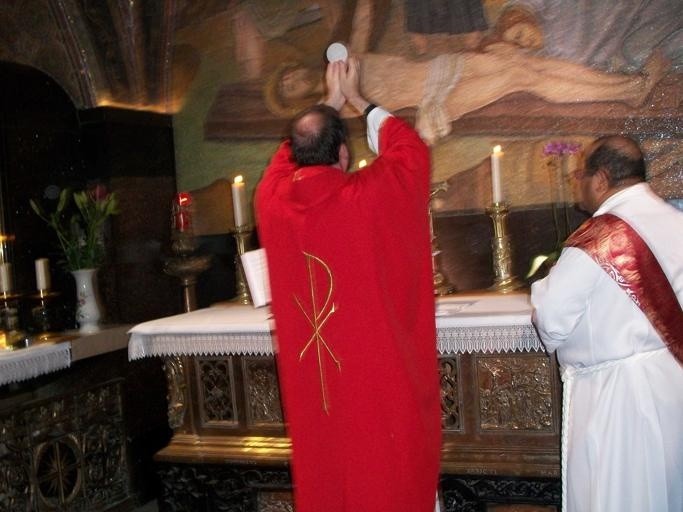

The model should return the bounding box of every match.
[526,134,683,512]
[253,42,443,512]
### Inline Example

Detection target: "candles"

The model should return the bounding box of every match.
[1,256,51,291]
[489,144,504,203]
[231,175,252,227]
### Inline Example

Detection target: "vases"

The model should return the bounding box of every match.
[68,264,103,336]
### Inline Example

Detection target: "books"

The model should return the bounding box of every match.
[239,247,272,309]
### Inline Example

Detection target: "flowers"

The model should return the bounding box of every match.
[35,182,124,270]
[539,140,585,244]
